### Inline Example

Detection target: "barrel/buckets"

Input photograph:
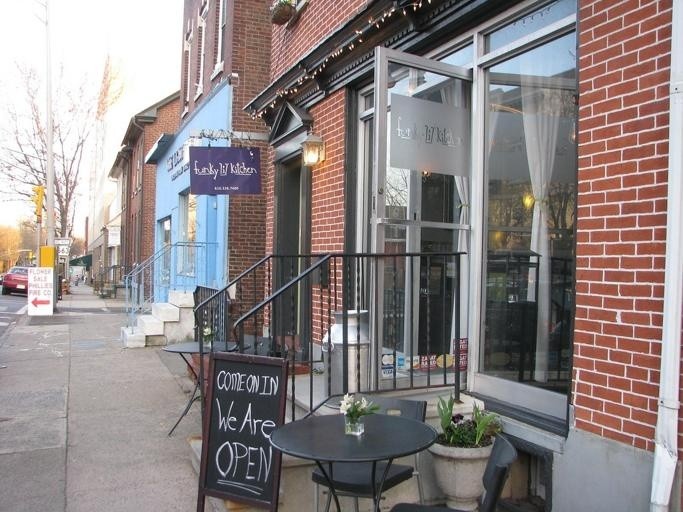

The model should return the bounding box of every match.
[319,307,371,408]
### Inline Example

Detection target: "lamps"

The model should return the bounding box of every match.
[301,124,324,168]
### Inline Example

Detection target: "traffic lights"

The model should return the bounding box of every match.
[31,186,43,206]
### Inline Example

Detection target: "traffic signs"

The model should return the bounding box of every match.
[28,267,54,316]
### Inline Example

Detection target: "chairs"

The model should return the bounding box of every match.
[312,392,515,511]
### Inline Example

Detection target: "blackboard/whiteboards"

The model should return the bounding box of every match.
[199,352,289,510]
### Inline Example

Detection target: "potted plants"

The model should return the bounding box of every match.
[269,0,296,25]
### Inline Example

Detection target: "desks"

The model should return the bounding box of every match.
[269,415,437,512]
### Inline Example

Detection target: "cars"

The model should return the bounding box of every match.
[2,266,27,294]
[0,272,6,285]
